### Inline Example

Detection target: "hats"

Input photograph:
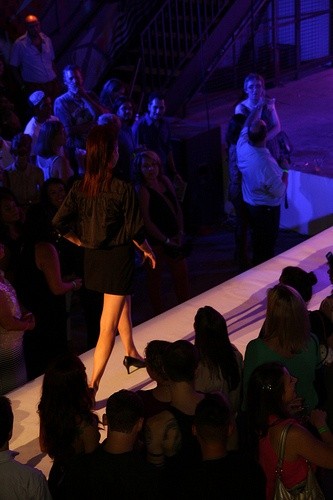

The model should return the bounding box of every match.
[29,90,45,105]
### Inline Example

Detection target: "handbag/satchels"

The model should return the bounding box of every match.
[315,310,333,366]
[273,423,325,500]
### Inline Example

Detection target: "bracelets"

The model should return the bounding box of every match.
[317,425,328,433]
[254,104,262,110]
[165,237,170,243]
[283,167,291,174]
[72,280,80,290]
[77,241,84,247]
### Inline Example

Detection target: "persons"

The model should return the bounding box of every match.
[0,0,333,500]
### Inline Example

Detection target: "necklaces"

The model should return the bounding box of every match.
[148,452,163,458]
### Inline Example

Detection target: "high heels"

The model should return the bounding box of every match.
[124,356,146,374]
